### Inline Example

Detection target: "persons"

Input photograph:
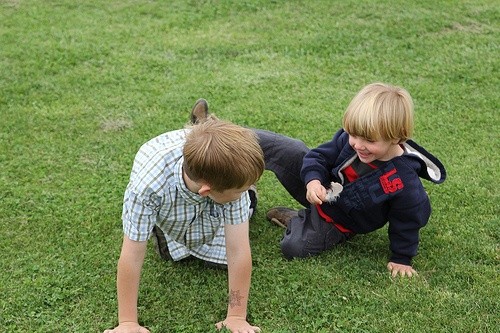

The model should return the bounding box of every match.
[191,78,448,276]
[104,121,267,333]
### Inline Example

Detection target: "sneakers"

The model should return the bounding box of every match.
[191,98,209,126]
[266,206,299,228]
